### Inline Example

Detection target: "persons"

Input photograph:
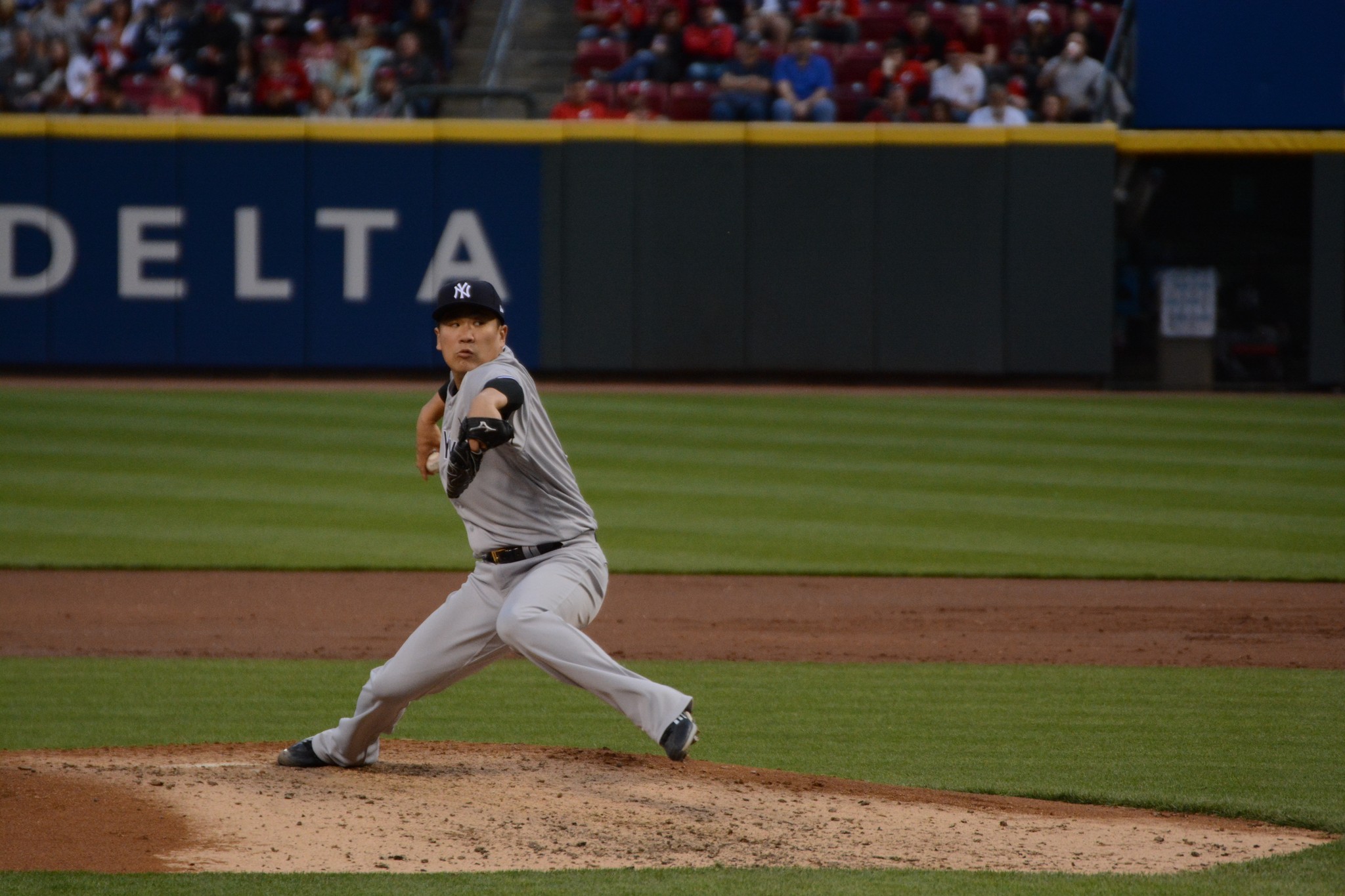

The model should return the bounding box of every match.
[278,278,701,769]
[0,0,463,119]
[547,0,1130,127]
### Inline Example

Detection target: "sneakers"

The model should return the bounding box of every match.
[661,709,701,762]
[278,736,327,768]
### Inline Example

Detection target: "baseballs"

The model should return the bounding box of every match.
[427,450,438,474]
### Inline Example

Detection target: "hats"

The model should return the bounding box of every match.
[431,280,506,323]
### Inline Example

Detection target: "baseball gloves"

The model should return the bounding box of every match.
[448,419,512,501]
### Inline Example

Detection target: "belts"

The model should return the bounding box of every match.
[484,542,563,564]
